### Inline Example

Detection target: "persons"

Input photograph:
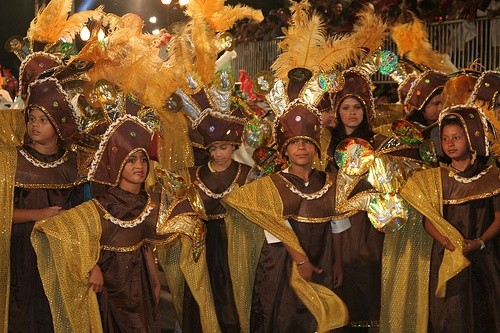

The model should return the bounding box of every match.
[312,67,398,333]
[1,76,101,333]
[1,1,500,198]
[27,113,184,333]
[219,97,434,333]
[167,106,272,332]
[398,104,500,333]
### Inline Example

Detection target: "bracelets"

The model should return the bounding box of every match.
[297,258,310,266]
[477,237,485,252]
[442,238,450,248]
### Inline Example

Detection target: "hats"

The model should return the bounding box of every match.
[332,67,376,122]
[438,104,489,158]
[18,51,65,103]
[25,78,84,142]
[188,107,246,149]
[315,93,332,112]
[397,71,421,104]
[274,97,323,158]
[466,70,500,111]
[88,113,155,186]
[404,71,449,110]
